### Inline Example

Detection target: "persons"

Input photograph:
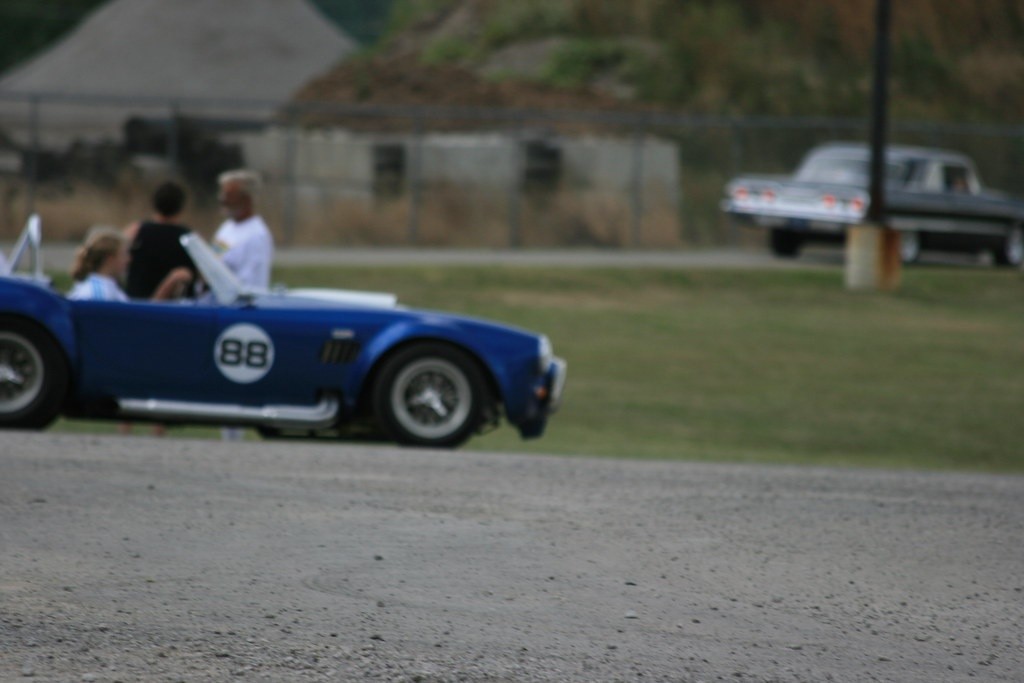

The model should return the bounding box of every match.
[66,167,273,439]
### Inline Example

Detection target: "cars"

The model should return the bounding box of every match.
[717,142,1023,267]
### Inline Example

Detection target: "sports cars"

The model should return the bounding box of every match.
[2,212,568,450]
